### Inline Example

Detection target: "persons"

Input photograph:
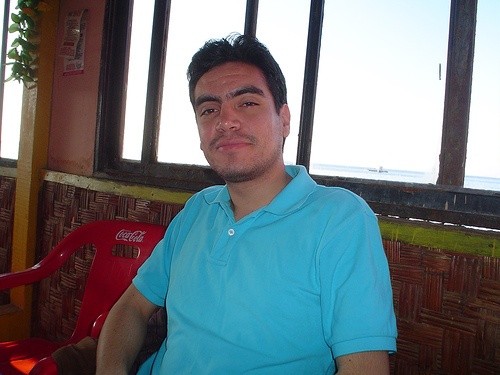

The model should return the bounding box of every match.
[96,33,399,375]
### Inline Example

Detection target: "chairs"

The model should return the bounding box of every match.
[0,220,164,375]
